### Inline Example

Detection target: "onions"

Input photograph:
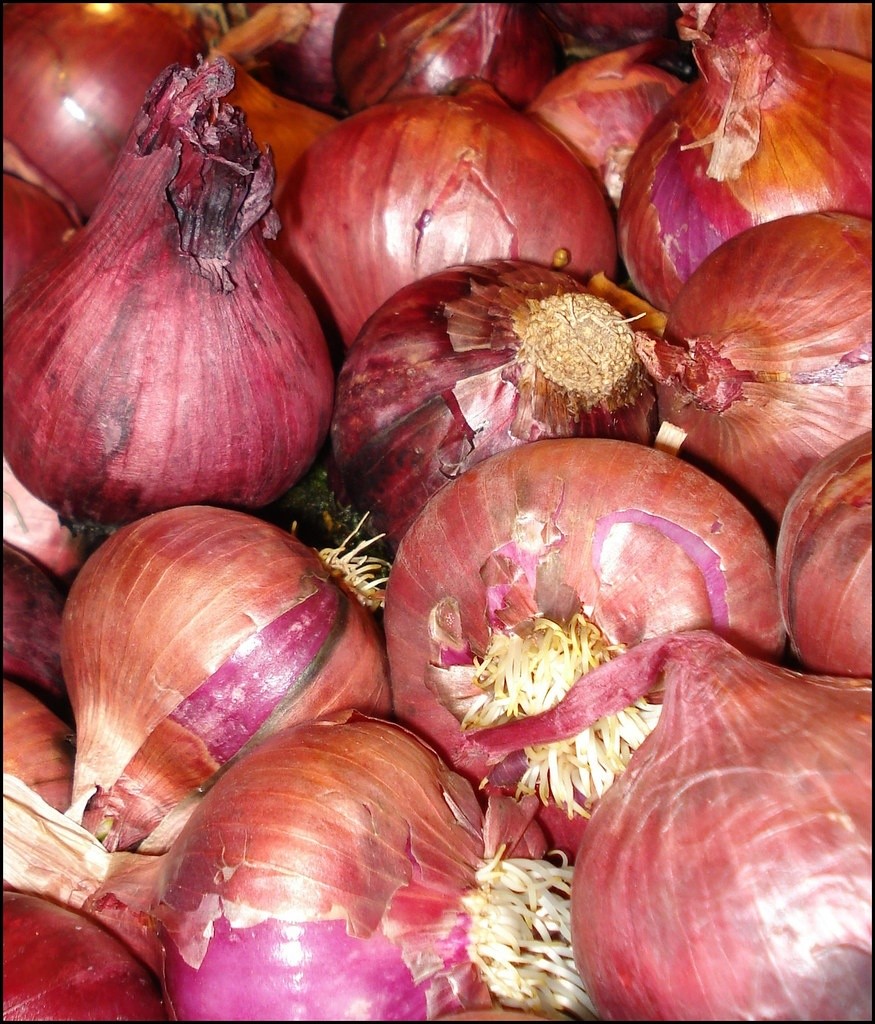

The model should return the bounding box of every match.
[0,2,875,1024]
